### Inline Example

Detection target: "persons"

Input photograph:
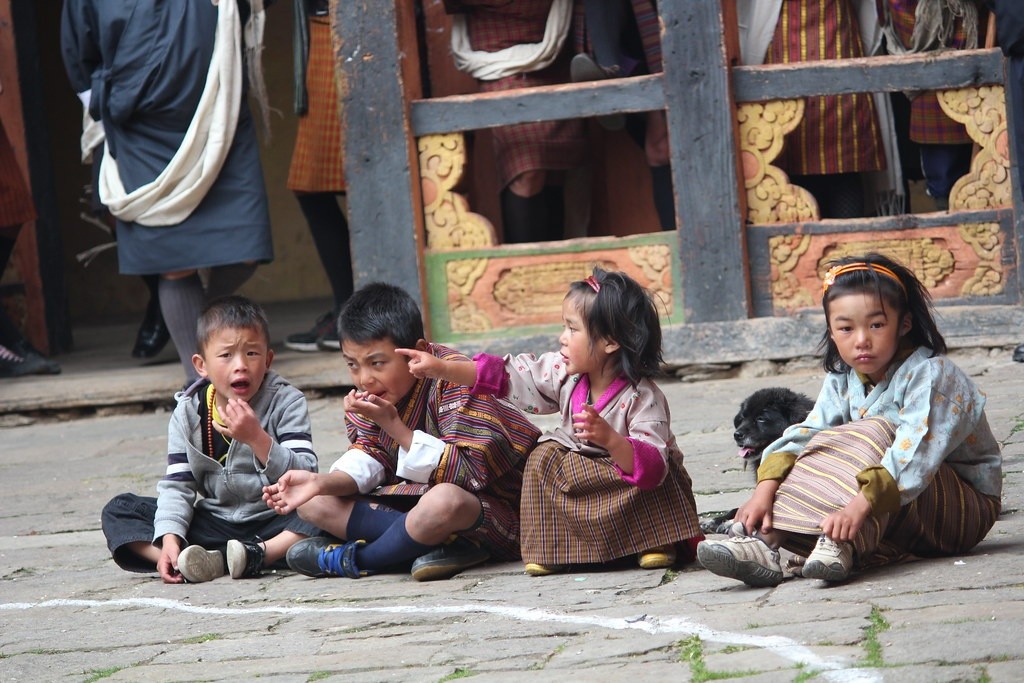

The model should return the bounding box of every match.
[101,297,324,584]
[262,281,542,582]
[0,0,1023,381]
[393,268,706,576]
[696,251,1005,588]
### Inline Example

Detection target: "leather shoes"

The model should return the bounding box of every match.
[133,296,171,358]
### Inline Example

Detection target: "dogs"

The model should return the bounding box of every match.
[732,387,817,482]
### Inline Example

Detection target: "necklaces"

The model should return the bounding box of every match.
[207,388,217,459]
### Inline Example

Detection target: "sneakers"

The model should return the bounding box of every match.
[283,310,335,351]
[411,534,492,582]
[0,329,61,378]
[697,521,783,587]
[226,535,267,579]
[170,545,224,584]
[316,316,342,351]
[802,531,855,583]
[286,537,377,579]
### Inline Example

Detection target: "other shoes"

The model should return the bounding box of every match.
[524,563,571,576]
[570,53,626,131]
[1013,343,1024,362]
[637,543,677,569]
